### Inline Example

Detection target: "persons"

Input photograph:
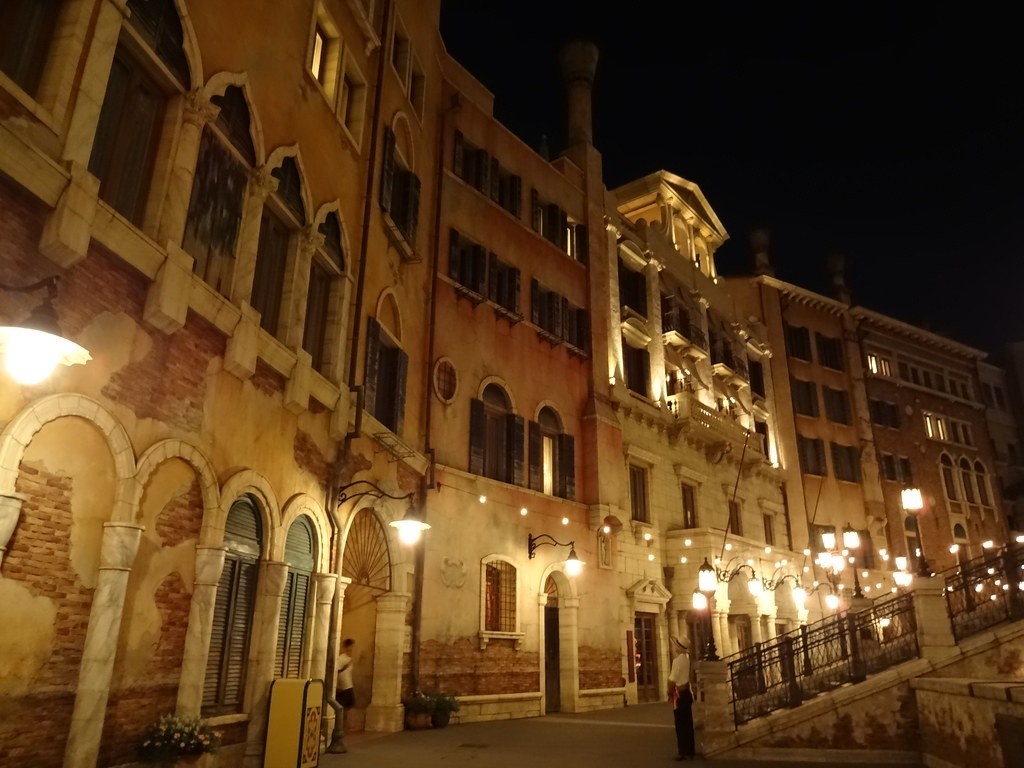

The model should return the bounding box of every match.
[335,638,356,737]
[667,634,697,762]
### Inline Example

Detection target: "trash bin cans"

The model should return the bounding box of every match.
[264,679,325,768]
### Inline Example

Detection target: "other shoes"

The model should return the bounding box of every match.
[674,753,693,762]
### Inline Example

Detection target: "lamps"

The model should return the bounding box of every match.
[528,533,586,574]
[803,582,838,609]
[693,586,708,610]
[337,480,431,546]
[0,274,93,387]
[762,566,806,604]
[715,555,761,597]
[697,557,716,591]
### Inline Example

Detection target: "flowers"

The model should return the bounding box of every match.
[135,712,225,761]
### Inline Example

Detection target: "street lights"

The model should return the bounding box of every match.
[843,520,866,599]
[690,556,720,662]
[900,479,931,577]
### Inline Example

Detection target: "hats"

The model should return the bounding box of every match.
[670,634,690,653]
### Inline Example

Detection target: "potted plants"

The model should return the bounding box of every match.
[416,688,461,729]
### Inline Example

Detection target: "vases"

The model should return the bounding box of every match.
[147,752,221,768]
